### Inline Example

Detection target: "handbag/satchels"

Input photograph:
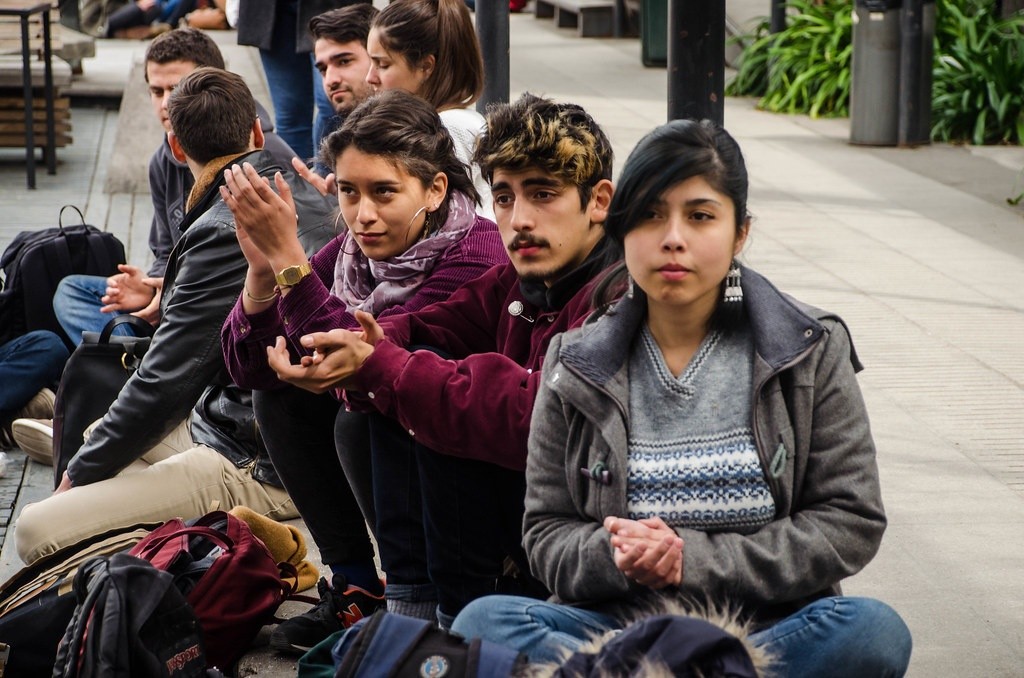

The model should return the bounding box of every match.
[53,313,156,491]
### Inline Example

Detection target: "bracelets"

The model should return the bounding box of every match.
[244,287,278,302]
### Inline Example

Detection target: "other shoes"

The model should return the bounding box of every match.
[2,384,54,448]
[13,415,52,466]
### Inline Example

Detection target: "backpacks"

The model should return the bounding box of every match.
[335,606,530,678]
[0,511,298,678]
[1,203,128,345]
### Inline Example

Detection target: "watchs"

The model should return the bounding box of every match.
[276,262,313,286]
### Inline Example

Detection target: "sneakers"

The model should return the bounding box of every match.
[271,573,388,653]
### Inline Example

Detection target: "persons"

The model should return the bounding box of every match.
[268,95,630,627]
[80,1,179,36]
[449,118,912,678]
[289,0,500,228]
[12,27,309,468]
[238,2,374,180]
[17,68,346,567]
[311,6,381,129]
[1,330,69,451]
[223,89,510,652]
